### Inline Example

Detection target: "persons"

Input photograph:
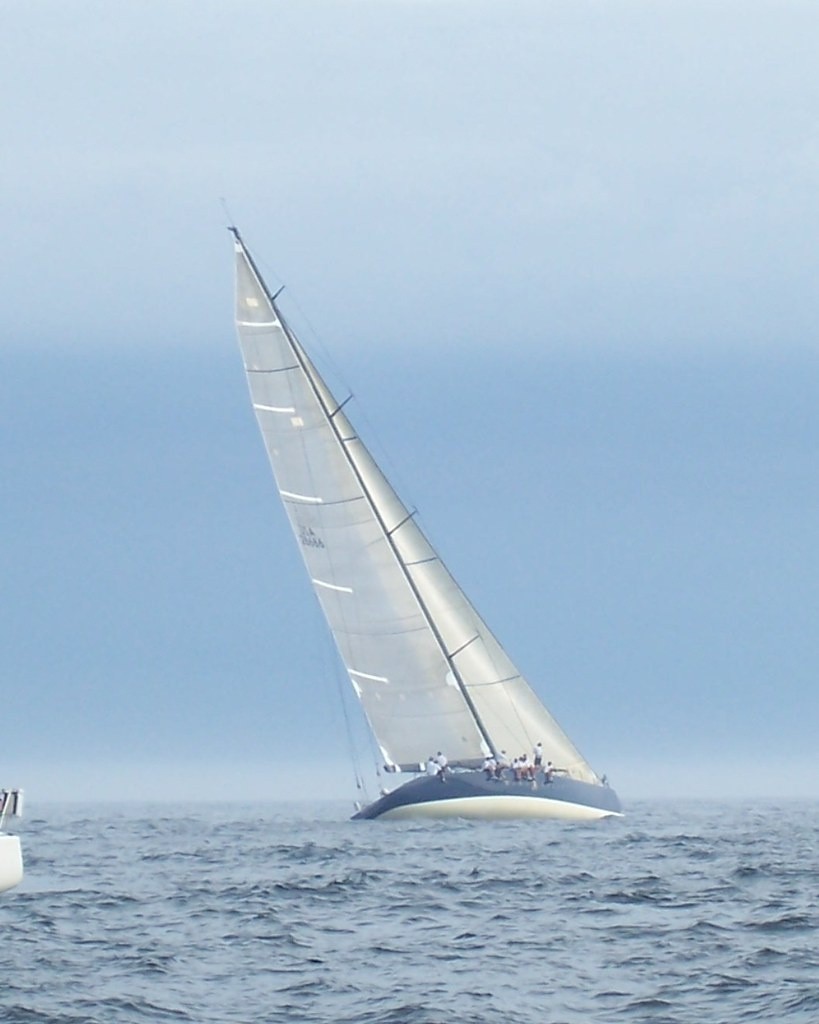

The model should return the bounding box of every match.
[482,743,555,782]
[426,752,456,783]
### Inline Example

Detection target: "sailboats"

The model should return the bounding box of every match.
[227,225,624,826]
